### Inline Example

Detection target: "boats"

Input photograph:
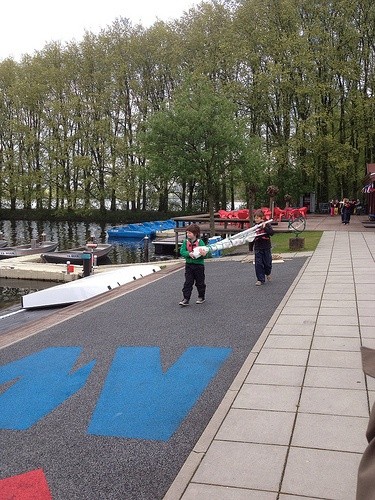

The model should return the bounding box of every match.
[0,231,59,260]
[40,234,112,265]
[106,219,184,237]
[0,239,8,248]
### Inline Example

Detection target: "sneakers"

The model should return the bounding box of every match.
[195,298,204,304]
[179,299,189,305]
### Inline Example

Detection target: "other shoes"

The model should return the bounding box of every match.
[265,275,272,281]
[255,281,263,286]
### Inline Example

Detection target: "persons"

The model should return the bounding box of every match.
[249,210,273,286]
[179,224,208,305]
[330,198,360,224]
